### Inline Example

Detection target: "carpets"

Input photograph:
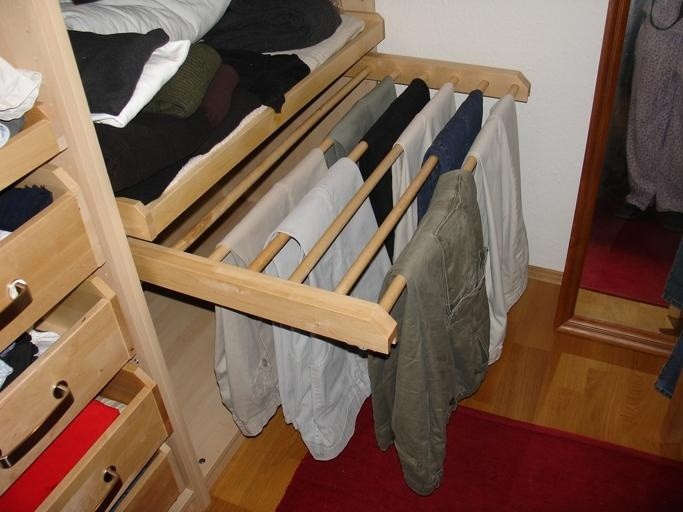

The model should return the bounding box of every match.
[273,397,683,512]
[579,196,683,308]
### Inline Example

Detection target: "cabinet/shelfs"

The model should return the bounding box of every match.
[2,103,212,512]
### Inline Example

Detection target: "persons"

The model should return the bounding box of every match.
[606,0,681,234]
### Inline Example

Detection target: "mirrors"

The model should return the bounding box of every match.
[550,0,682,357]
